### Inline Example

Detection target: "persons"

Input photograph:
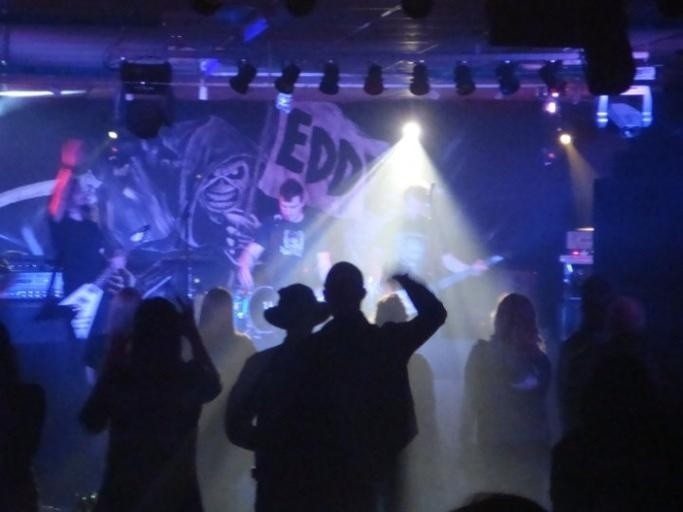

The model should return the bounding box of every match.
[2,323,48,510]
[555,273,660,511]
[454,492,548,511]
[95,287,141,375]
[609,297,681,511]
[78,296,224,512]
[457,293,553,511]
[236,179,333,287]
[389,185,491,277]
[374,292,442,511]
[317,263,448,512]
[197,288,258,511]
[46,179,112,372]
[224,283,333,511]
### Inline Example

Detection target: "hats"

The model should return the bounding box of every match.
[264,284,329,329]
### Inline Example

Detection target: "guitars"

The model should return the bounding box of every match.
[37,225,151,339]
[376,255,503,318]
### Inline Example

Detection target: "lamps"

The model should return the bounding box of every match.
[231,56,566,95]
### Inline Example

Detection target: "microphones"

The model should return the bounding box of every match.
[273,214,282,228]
[425,183,435,208]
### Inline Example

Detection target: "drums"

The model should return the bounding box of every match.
[234,282,280,333]
